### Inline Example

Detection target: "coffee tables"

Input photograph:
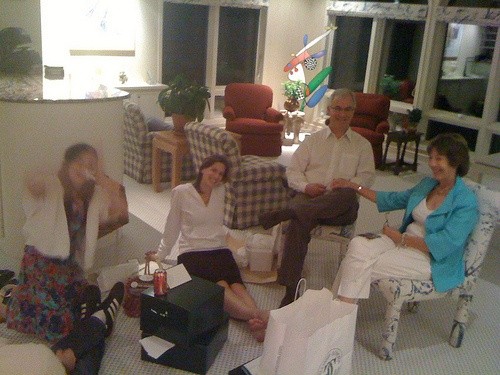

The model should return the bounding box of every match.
[281,110,305,144]
[380,132,422,175]
[152,130,188,193]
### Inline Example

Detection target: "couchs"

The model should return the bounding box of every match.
[123,100,195,183]
[223,83,284,157]
[326,93,391,169]
[186,122,289,229]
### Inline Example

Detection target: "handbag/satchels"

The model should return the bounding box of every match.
[261,278,358,375]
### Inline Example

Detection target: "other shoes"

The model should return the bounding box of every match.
[260,210,283,229]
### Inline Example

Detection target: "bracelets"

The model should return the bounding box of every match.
[400,232,408,246]
[358,185,362,192]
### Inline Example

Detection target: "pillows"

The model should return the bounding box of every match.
[142,108,172,131]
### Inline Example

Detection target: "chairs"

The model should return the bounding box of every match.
[273,221,357,275]
[371,178,500,361]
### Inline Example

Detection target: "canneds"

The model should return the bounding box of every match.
[154,269,168,296]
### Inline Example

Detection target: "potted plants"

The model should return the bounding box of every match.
[403,108,422,132]
[283,80,309,112]
[158,73,211,136]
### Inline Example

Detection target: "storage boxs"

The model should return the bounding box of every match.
[248,234,273,272]
[140,275,229,375]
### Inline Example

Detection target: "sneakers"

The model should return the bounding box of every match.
[81,285,101,319]
[99,281,124,337]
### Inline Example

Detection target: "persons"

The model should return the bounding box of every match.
[0,142,129,345]
[0,282,124,375]
[144,155,269,342]
[331,133,478,305]
[250,88,375,308]
